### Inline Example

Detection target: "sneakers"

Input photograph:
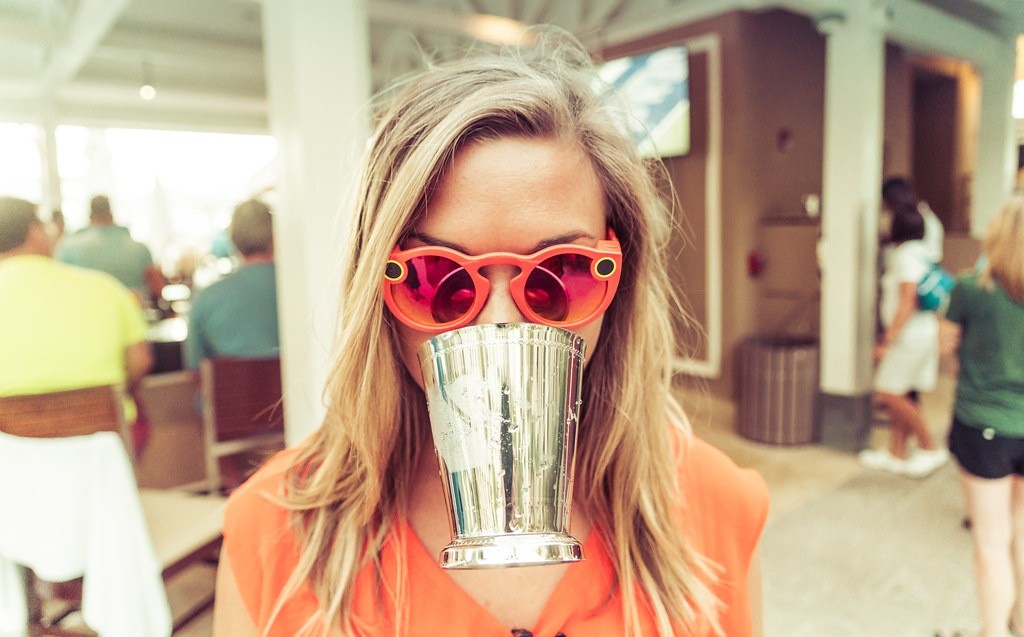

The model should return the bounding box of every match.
[858,446,949,481]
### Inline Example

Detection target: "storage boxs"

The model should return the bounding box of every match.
[734,333,820,444]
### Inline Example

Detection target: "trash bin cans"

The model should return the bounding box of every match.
[733,333,820,444]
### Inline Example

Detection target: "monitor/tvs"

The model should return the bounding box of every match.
[584,42,690,159]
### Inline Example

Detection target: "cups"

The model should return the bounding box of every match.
[415,322,587,572]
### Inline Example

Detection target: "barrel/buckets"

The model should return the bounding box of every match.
[738,336,819,445]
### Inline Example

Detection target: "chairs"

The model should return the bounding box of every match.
[0,432,229,637]
[0,386,141,491]
[199,356,286,497]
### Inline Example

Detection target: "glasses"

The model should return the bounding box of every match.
[380,225,624,334]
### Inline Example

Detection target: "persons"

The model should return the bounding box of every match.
[209,23,771,637]
[882,174,944,266]
[48,210,65,237]
[935,196,1024,636]
[0,196,155,466]
[857,202,949,480]
[178,199,278,370]
[52,196,153,289]
